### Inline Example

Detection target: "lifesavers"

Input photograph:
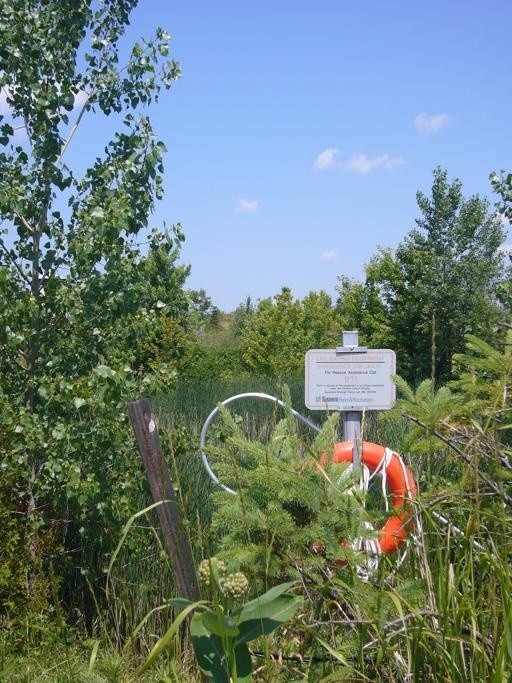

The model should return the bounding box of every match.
[305,440,417,564]
